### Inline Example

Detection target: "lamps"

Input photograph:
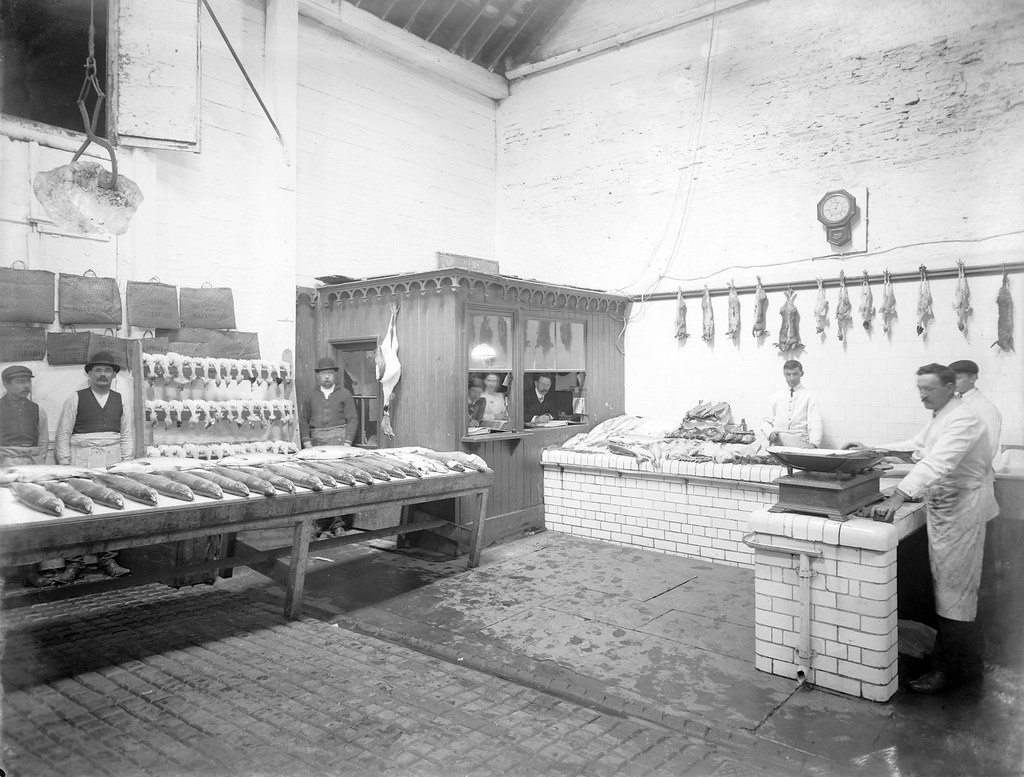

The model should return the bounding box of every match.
[472,344,497,360]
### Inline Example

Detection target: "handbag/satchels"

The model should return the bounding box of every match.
[0,321,47,362]
[0,260,56,324]
[127,327,261,378]
[58,269,122,325]
[88,327,127,369]
[46,324,90,364]
[125,277,180,330]
[180,283,237,330]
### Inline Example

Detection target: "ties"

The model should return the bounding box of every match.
[790,388,794,397]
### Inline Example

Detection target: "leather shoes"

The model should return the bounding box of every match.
[52,566,86,584]
[96,560,130,578]
[25,571,51,587]
[908,668,967,695]
[334,526,346,537]
[918,643,971,665]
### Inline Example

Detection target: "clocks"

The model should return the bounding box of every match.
[818,189,857,247]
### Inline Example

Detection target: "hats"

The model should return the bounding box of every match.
[84,351,121,373]
[948,360,979,373]
[2,365,35,382]
[314,357,339,372]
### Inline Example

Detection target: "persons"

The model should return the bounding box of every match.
[523,373,561,427]
[297,357,359,542]
[466,371,507,435]
[945,358,1003,467]
[0,365,53,588]
[871,362,998,694]
[765,359,823,450]
[53,350,131,584]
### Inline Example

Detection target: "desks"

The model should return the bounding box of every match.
[1,447,494,621]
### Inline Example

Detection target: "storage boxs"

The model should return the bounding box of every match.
[116,533,222,592]
